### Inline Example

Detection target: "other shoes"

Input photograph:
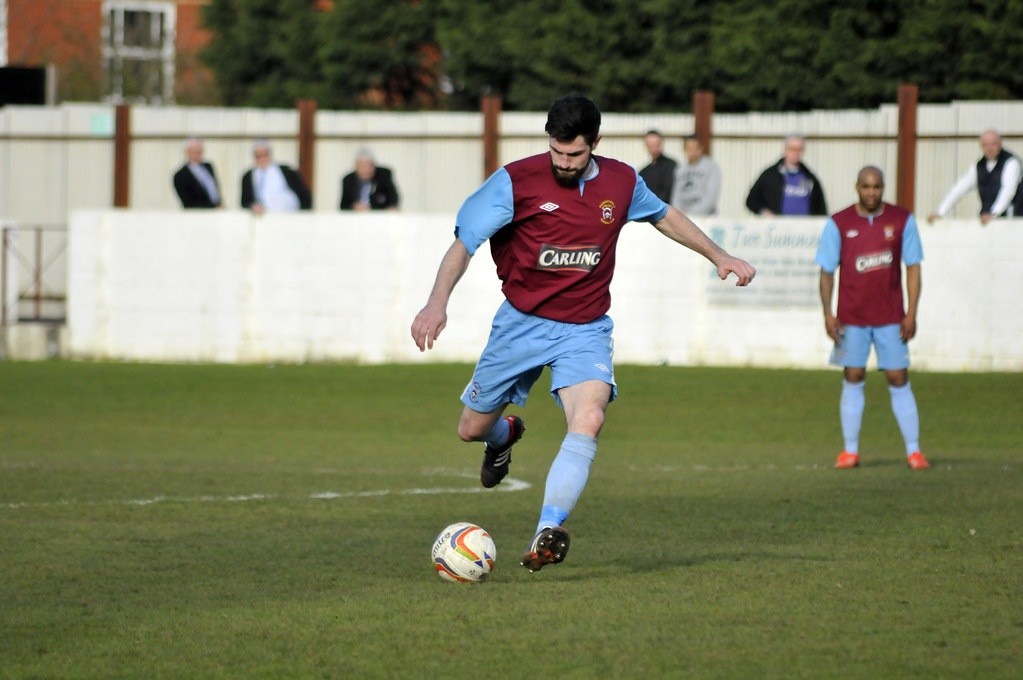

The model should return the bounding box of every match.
[837,451,859,469]
[909,453,929,470]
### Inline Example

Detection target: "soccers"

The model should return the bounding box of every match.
[431,521,499,583]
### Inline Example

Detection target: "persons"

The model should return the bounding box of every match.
[173,141,223,209]
[340,148,399,212]
[241,141,313,214]
[410,95,757,574]
[746,134,827,217]
[637,131,677,203]
[817,166,932,471]
[926,131,1023,222]
[671,136,721,219]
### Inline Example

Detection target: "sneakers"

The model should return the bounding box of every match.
[520,526,570,572]
[480,415,524,488]
